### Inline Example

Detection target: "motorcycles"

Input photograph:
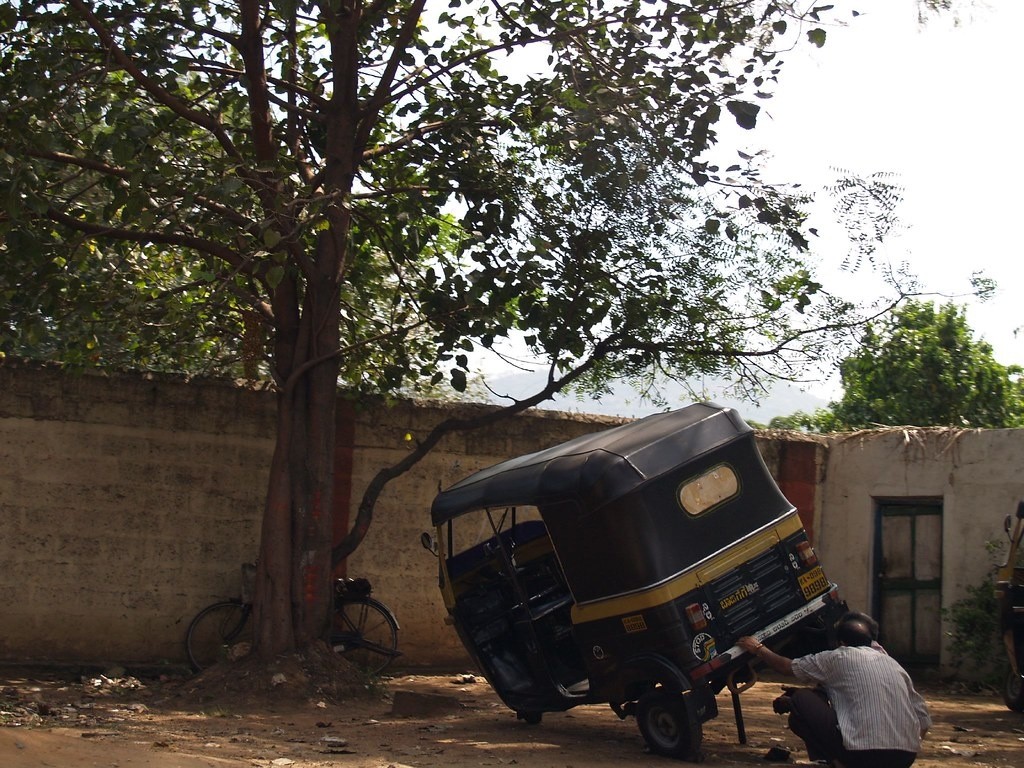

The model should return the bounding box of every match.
[990,501,1024,713]
[420,399,850,761]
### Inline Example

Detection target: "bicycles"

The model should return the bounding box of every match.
[185,561,403,678]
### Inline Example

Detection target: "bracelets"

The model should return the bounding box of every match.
[752,644,765,654]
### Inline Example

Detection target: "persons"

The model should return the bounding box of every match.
[735,612,922,768]
[773,613,930,739]
